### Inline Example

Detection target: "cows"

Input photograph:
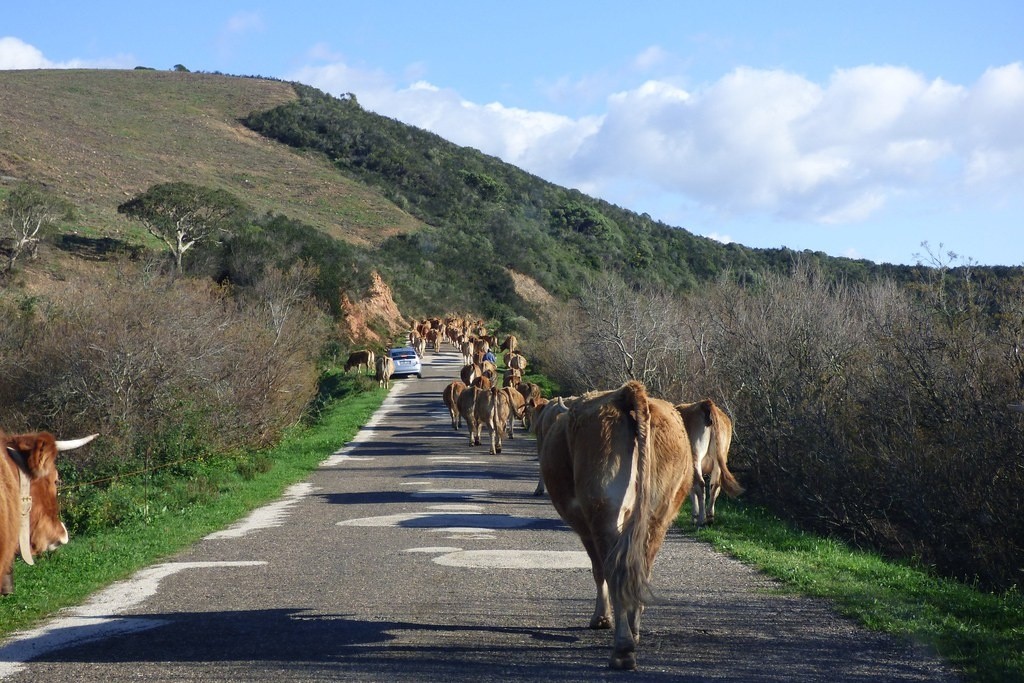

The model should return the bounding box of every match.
[0,427,101,596]
[342,312,748,671]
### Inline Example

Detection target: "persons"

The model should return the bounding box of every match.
[482,349,496,363]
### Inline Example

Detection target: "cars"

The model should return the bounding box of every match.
[388,348,423,378]
